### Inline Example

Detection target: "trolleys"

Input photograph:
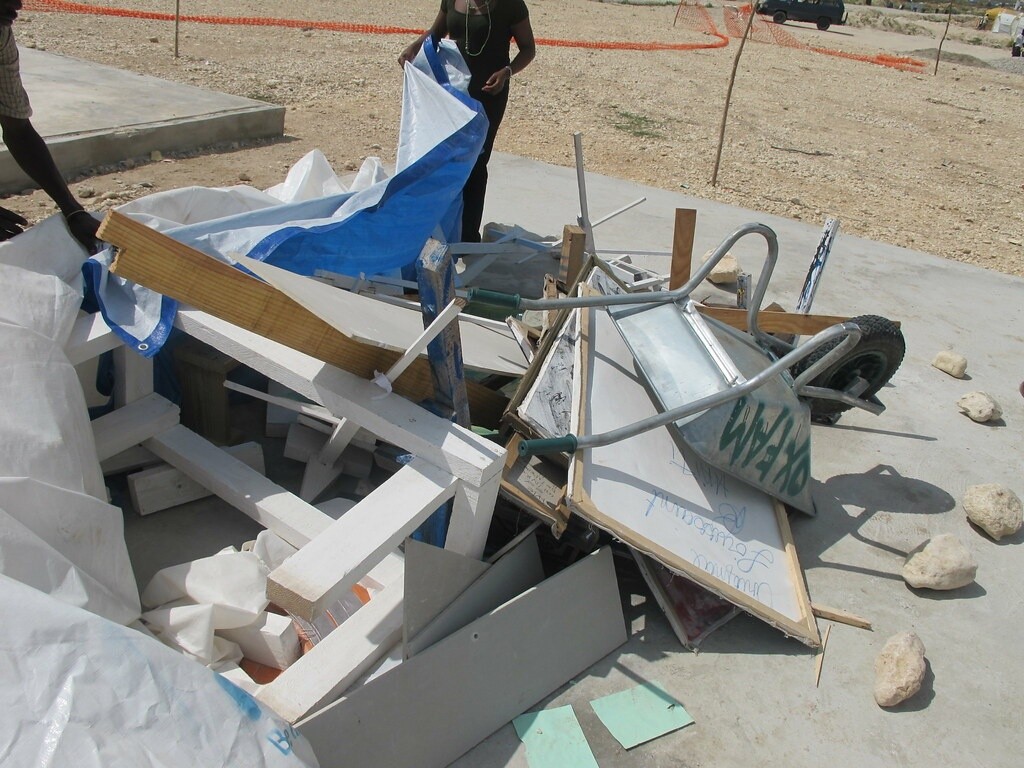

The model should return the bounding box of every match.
[466,223,905,456]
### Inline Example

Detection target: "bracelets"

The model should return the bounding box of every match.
[505,66,512,79]
[66,210,85,219]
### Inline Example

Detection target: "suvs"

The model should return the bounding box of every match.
[754,0,848,30]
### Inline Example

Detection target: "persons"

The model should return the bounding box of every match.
[0,0,102,256]
[977,13,989,30]
[398,0,536,258]
[1012,29,1024,57]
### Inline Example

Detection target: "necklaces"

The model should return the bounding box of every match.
[465,0,492,56]
